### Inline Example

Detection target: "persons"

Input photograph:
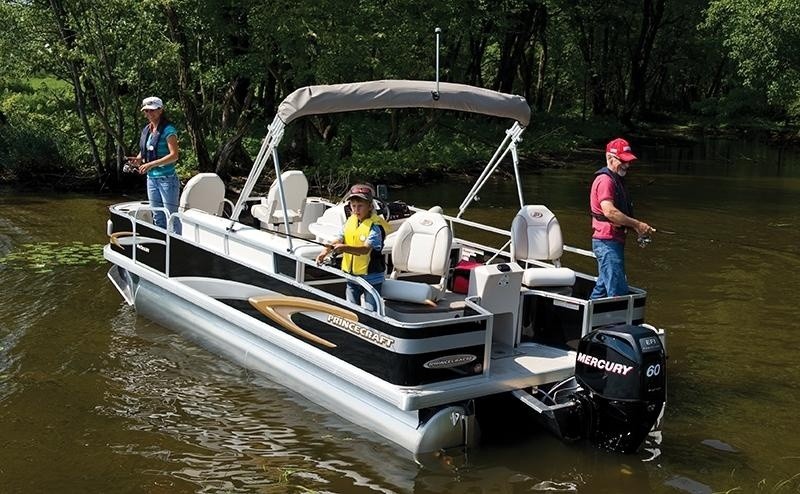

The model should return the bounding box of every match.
[313,184,388,312]
[124,95,185,238]
[587,136,656,298]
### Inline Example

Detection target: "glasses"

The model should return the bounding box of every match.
[349,187,372,201]
[612,155,633,165]
[140,100,163,108]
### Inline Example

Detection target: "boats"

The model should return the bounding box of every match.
[102,25,671,457]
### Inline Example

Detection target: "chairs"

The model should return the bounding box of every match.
[252,170,309,233]
[380,209,454,308]
[178,170,226,221]
[507,202,576,289]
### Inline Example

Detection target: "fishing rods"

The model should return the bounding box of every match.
[30,117,139,175]
[649,227,799,256]
[123,193,334,251]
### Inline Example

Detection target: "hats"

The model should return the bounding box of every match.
[345,184,375,202]
[606,138,639,162]
[140,97,163,112]
